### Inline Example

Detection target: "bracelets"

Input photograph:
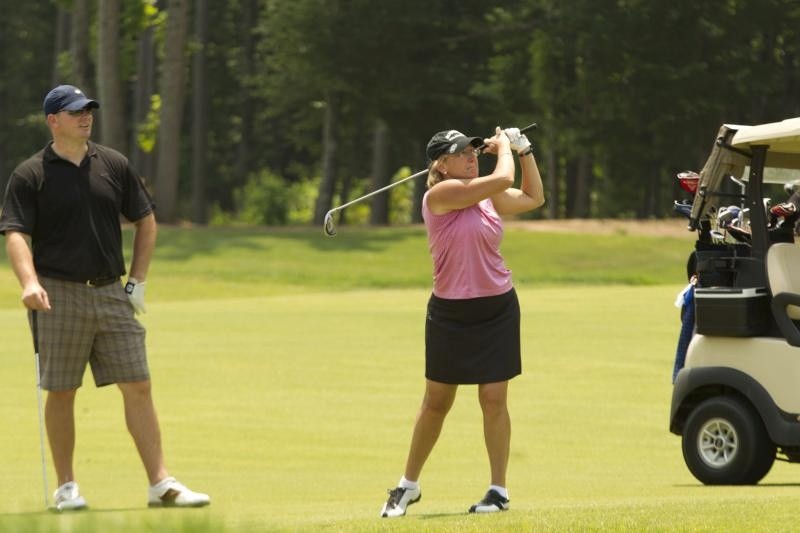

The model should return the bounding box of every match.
[518,150,532,157]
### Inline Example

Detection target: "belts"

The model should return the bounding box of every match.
[80,278,116,288]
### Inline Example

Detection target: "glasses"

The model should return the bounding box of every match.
[56,106,91,116]
[443,148,480,160]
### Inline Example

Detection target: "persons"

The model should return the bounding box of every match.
[383,127,545,518]
[0,86,211,512]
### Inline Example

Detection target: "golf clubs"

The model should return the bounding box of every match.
[709,174,798,244]
[322,121,539,239]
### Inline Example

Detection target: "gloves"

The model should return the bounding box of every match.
[504,128,532,153]
[125,277,146,316]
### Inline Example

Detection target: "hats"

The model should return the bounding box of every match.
[43,85,99,114]
[427,129,484,161]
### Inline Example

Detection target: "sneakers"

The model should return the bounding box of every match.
[148,478,209,507]
[54,481,84,510]
[381,487,421,518]
[468,489,509,513]
